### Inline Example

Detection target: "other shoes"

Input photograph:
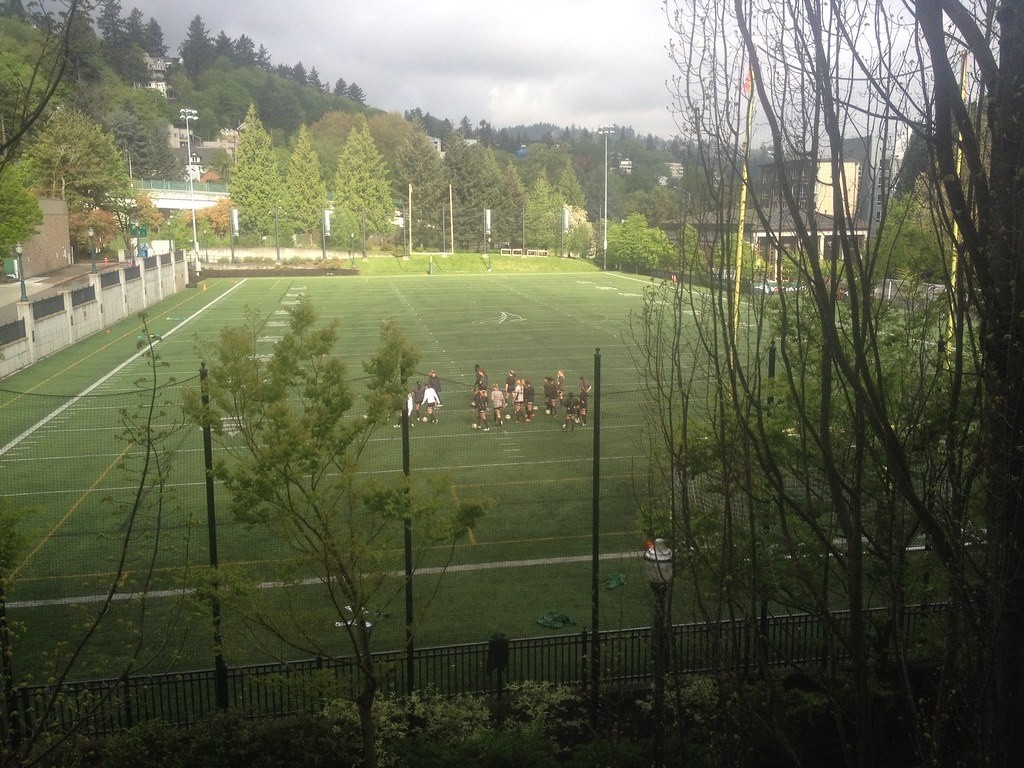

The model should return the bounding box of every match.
[525,416,531,422]
[581,423,587,427]
[530,412,535,416]
[409,423,414,427]
[393,424,400,428]
[553,413,558,418]
[476,425,481,428]
[483,428,490,431]
[563,428,571,432]
[431,419,439,423]
[496,423,503,427]
[516,419,521,424]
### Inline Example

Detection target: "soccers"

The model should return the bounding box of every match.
[533,405,539,412]
[574,418,579,424]
[561,424,567,430]
[422,416,429,423]
[471,422,478,430]
[544,409,551,415]
[504,413,511,421]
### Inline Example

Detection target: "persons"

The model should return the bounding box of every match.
[470,363,592,432]
[672,273,677,285]
[393,371,444,428]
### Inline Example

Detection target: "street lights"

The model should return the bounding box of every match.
[351,232,355,265]
[15,241,28,302]
[487,236,492,270]
[204,230,209,263]
[597,124,615,273]
[644,538,675,716]
[178,108,201,279]
[88,226,99,275]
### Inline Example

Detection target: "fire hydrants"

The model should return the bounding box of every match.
[103,257,110,267]
